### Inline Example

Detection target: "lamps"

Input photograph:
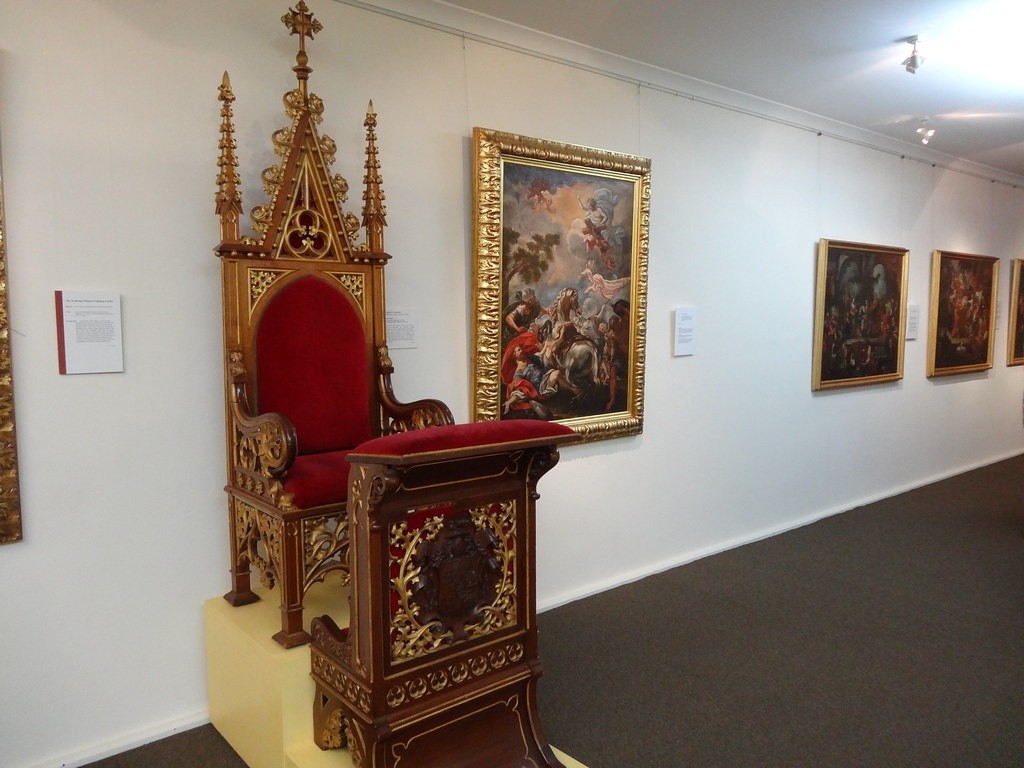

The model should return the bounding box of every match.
[900,34,927,75]
[914,117,939,147]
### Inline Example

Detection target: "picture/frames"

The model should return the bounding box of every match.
[1005,258,1024,368]
[925,248,1002,379]
[810,237,912,394]
[466,125,653,450]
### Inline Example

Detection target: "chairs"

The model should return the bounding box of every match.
[213,0,462,648]
[306,416,586,768]
